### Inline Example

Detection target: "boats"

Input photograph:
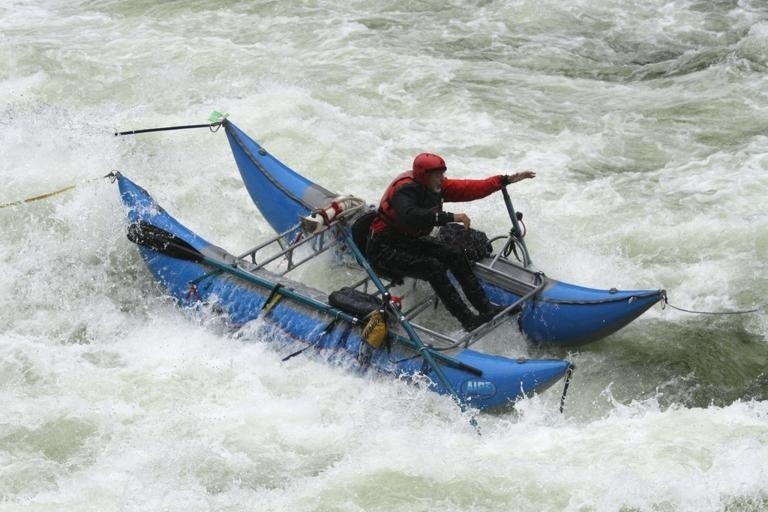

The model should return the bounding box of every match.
[111,109,667,415]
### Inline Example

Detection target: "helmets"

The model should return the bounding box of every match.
[412,152,447,177]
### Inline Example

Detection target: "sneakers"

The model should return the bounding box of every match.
[463,302,524,332]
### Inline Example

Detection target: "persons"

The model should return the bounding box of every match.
[370,154,538,331]
